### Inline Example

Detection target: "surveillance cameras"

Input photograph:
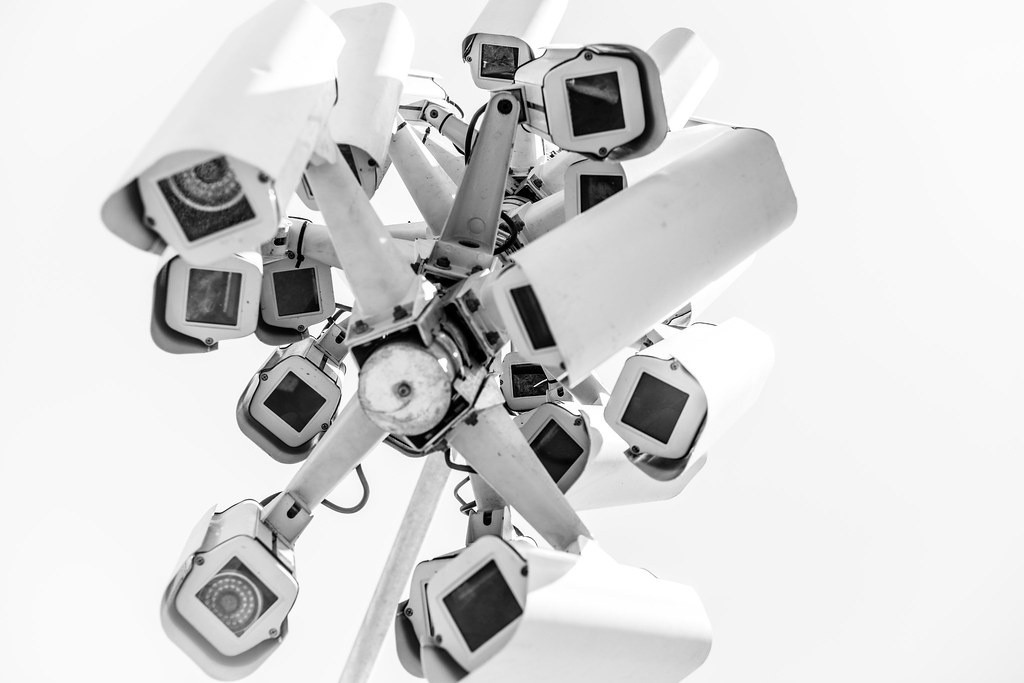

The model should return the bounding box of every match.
[103,0,796,683]
